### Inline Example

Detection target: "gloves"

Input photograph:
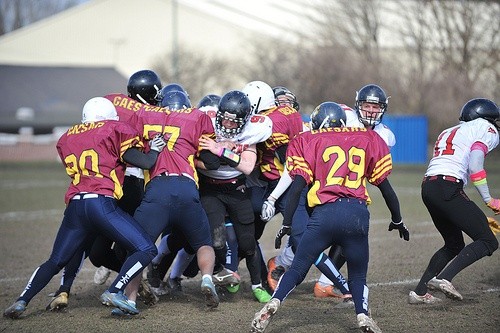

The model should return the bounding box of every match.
[150,133,166,152]
[486,198,500,236]
[388,221,409,241]
[259,196,277,222]
[274,226,292,249]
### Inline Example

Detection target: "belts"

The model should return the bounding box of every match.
[338,198,366,205]
[156,173,195,182]
[72,194,114,199]
[423,176,460,183]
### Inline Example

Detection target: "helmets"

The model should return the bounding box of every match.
[81,97,119,125]
[197,80,299,123]
[127,70,191,112]
[458,98,500,122]
[354,83,391,105]
[310,102,347,130]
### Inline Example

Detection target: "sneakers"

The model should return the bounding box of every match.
[249,298,280,333]
[46,292,68,312]
[3,300,26,319]
[93,265,111,286]
[356,313,383,333]
[313,282,355,310]
[250,255,286,303]
[408,276,464,304]
[99,277,183,318]
[200,269,240,309]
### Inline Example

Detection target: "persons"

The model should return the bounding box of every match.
[3,69,410,333]
[411,98,500,306]
